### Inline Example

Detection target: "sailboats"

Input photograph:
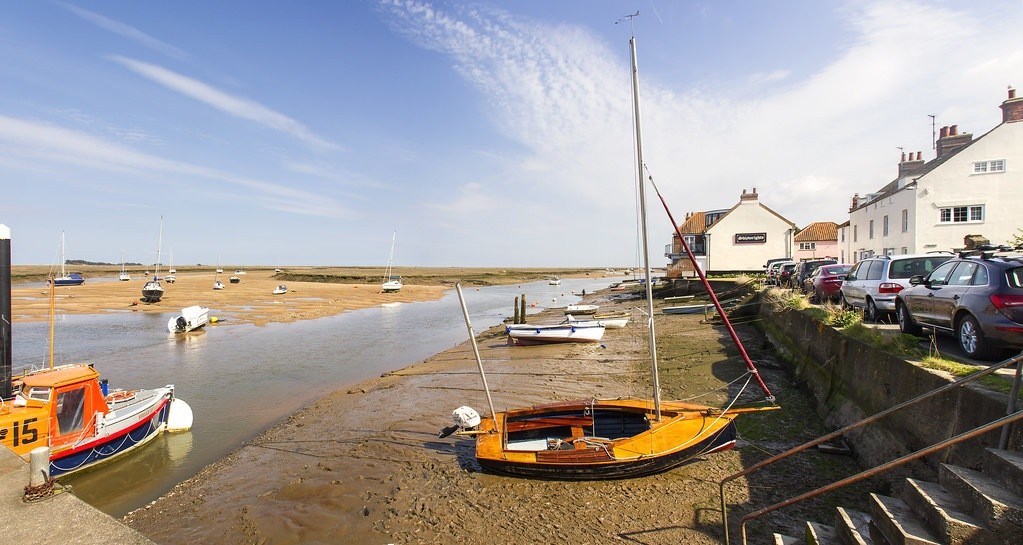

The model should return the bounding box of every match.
[45,230,85,287]
[381,228,403,293]
[163,246,177,283]
[169,252,176,274]
[118,247,131,281]
[215,250,224,273]
[141,215,165,302]
[436,10,784,484]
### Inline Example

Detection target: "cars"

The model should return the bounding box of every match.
[763,257,855,304]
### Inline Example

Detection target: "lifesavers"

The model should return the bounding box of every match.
[105,390,136,402]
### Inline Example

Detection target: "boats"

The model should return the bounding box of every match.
[564,303,600,315]
[272,284,288,295]
[661,303,715,315]
[274,265,281,272]
[167,305,210,333]
[503,320,608,343]
[663,293,695,303]
[591,308,633,319]
[229,276,240,283]
[213,279,225,289]
[0,273,176,479]
[234,270,247,275]
[609,276,669,291]
[548,274,561,285]
[564,312,631,329]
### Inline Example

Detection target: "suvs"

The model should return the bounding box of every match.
[837,249,958,323]
[893,241,1023,362]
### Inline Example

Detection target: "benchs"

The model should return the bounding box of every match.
[571,425,587,450]
[507,415,593,432]
